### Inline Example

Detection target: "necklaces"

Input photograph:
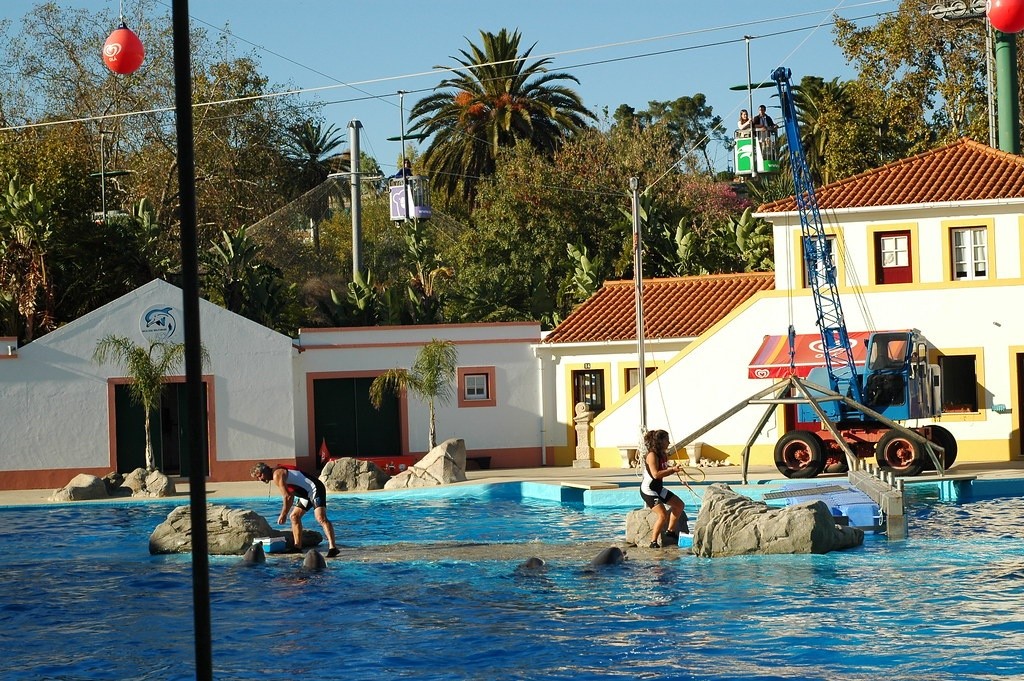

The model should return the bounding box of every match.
[268,468,271,501]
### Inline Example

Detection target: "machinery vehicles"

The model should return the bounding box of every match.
[723,66,958,480]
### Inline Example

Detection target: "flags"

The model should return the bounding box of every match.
[319,438,330,463]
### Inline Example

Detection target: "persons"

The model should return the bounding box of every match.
[395,159,413,197]
[738,105,775,150]
[639,429,684,549]
[250,462,340,558]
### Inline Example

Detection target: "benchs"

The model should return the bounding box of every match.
[466,456,493,469]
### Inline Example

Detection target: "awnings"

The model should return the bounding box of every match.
[749,329,911,379]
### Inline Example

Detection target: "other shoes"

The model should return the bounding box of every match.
[650,541,660,548]
[325,547,340,558]
[286,546,303,554]
[665,531,678,539]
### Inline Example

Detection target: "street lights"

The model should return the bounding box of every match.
[628,172,649,438]
